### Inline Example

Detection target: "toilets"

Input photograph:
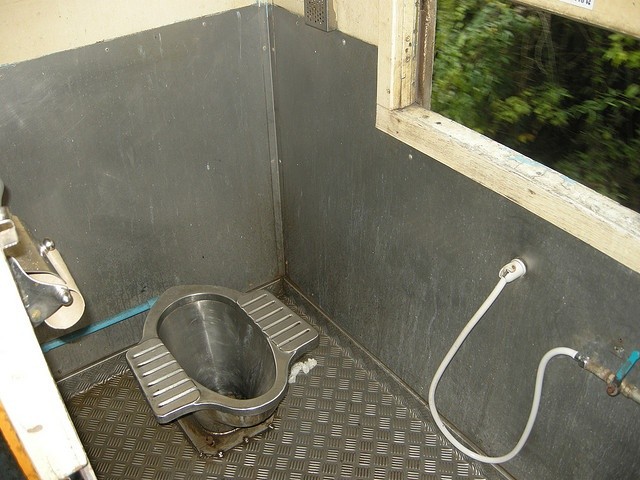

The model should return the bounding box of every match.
[127,285,320,426]
